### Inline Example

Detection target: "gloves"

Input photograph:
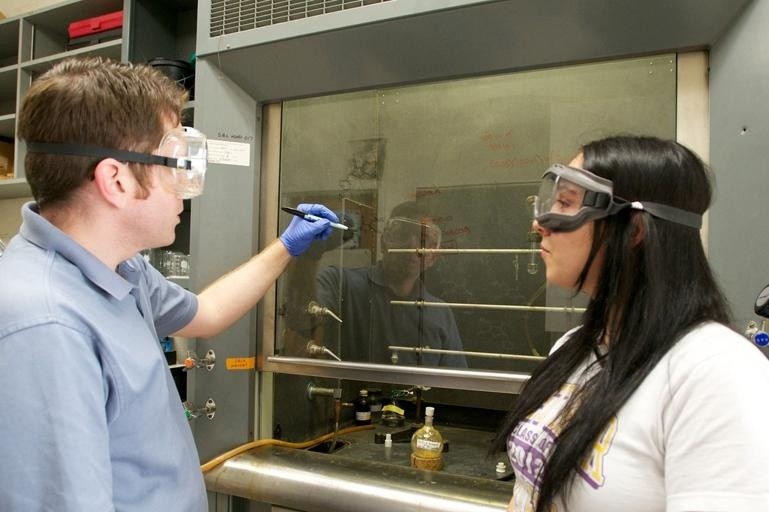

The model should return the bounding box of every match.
[278,202,341,257]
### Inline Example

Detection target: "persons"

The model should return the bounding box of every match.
[0,56,339,512]
[280,199,466,368]
[485,132,768,512]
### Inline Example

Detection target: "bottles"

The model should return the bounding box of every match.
[410,406,444,459]
[354,388,372,426]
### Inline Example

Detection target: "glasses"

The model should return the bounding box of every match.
[123,127,208,201]
[381,216,443,259]
[536,164,638,232]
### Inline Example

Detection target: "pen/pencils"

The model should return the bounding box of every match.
[281,207,351,231]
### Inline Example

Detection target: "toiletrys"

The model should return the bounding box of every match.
[412,408,444,471]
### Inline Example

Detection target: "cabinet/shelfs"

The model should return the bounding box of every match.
[0,0,173,256]
